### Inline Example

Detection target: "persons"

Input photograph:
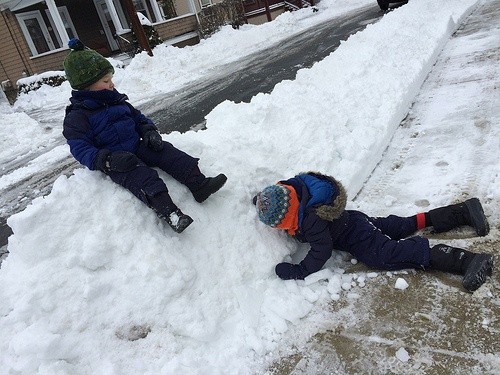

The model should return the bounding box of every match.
[253,170,493,292]
[62,38,228,233]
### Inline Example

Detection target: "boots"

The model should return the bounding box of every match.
[150,192,194,234]
[427,198,490,236]
[182,165,228,203]
[428,243,493,292]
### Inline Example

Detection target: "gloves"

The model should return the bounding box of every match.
[96,152,138,174]
[274,262,308,281]
[142,125,162,148]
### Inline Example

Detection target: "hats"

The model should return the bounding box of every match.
[62,39,115,89]
[251,183,298,236]
